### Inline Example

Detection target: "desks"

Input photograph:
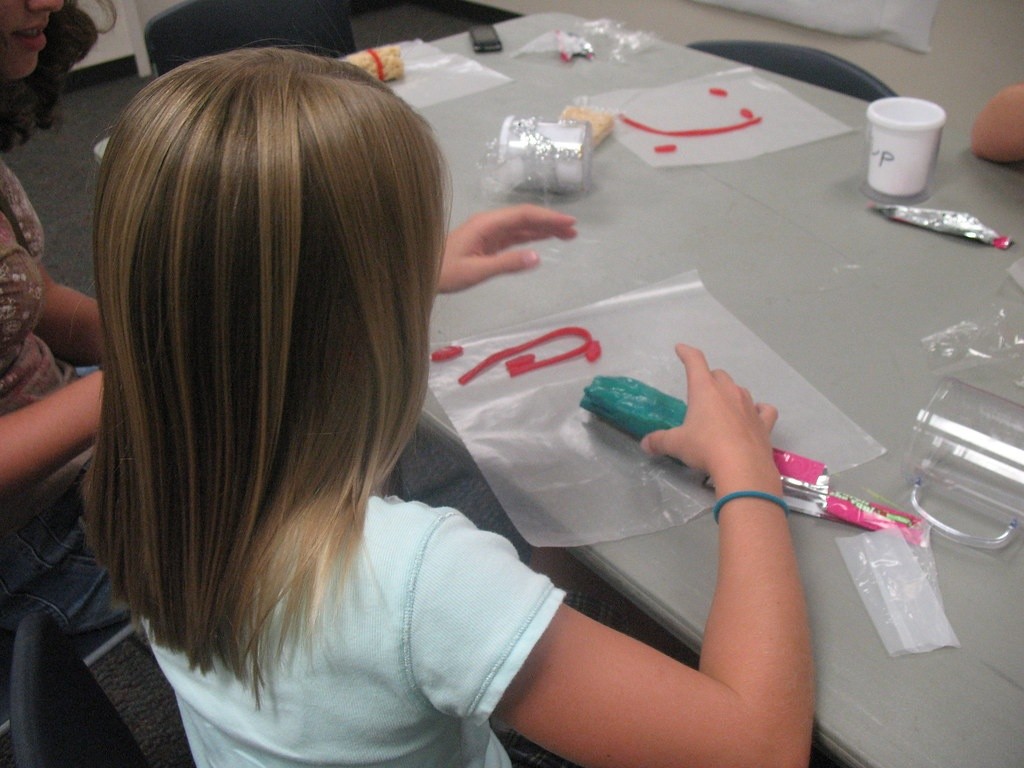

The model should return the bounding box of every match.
[92,13,1024,768]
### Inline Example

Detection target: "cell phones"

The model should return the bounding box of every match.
[469,23,502,52]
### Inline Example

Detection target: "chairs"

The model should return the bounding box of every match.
[142,0,357,78]
[686,42,897,102]
[0,610,149,768]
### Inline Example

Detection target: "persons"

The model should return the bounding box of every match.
[80,51,817,768]
[0,1,576,642]
[971,84,1024,162]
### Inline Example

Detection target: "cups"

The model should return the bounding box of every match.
[498,117,595,199]
[863,96,947,207]
[900,375,1024,549]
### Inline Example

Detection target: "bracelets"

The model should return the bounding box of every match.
[713,490,789,524]
